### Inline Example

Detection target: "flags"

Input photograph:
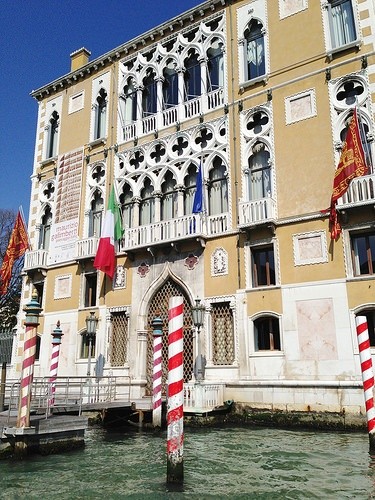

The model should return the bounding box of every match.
[0,208,31,295]
[189,159,202,231]
[93,183,124,281]
[321,105,367,240]
